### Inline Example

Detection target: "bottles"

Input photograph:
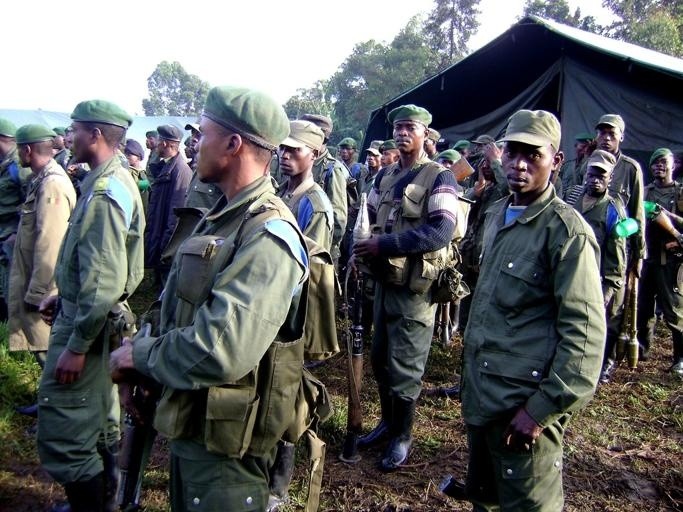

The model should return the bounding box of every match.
[353,193,371,241]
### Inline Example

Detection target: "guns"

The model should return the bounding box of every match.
[108,323,157,512]
[334,262,374,462]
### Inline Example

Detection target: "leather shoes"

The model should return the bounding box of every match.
[16,403,38,433]
[438,383,461,398]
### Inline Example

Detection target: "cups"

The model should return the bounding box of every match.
[449,157,476,181]
[616,200,655,237]
[138,179,149,190]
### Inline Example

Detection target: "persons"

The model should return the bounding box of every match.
[463,109,607,510]
[110,85,336,511]
[1,100,198,510]
[269,113,682,472]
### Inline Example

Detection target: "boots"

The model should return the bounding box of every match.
[451,303,460,333]
[600,329,683,384]
[266,446,296,511]
[55,440,122,512]
[358,389,416,471]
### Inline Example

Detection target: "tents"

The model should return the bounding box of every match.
[353,12,682,185]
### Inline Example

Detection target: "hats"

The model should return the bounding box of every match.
[649,148,671,169]
[126,118,201,161]
[387,105,495,163]
[576,114,625,173]
[338,137,396,156]
[201,84,290,152]
[496,110,562,152]
[0,118,74,144]
[71,99,133,129]
[280,113,333,150]
